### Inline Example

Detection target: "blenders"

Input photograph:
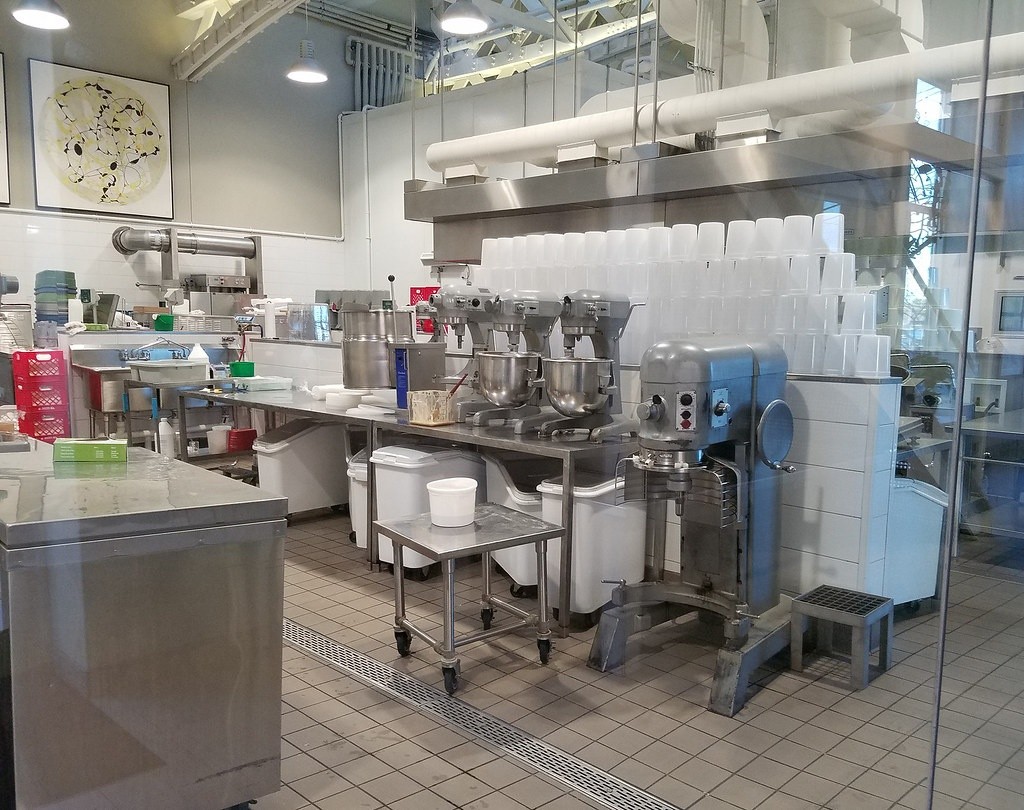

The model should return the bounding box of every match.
[538,288,646,444]
[471,288,562,434]
[429,286,495,422]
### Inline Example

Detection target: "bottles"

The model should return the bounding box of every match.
[155,418,174,458]
[188,343,210,379]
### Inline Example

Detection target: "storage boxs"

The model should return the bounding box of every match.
[253,418,647,629]
[12,349,71,446]
[154,313,174,331]
[34,270,78,327]
[228,361,254,377]
[882,479,949,614]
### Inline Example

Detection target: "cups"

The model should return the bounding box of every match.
[481,212,844,267]
[187,441,199,455]
[473,254,856,298]
[553,294,876,335]
[447,331,890,377]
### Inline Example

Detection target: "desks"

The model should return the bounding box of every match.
[944,411,1024,558]
[0,448,283,810]
[371,501,566,693]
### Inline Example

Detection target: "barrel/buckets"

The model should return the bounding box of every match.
[230,362,254,377]
[341,313,412,389]
[154,313,174,331]
[288,303,332,340]
[206,425,232,454]
[427,478,478,528]
[407,390,457,426]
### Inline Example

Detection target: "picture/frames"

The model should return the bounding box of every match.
[27,57,174,221]
[0,52,12,205]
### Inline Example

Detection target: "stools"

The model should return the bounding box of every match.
[789,584,893,689]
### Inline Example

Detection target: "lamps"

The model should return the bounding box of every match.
[437,0,490,34]
[11,0,70,30]
[287,0,329,83]
